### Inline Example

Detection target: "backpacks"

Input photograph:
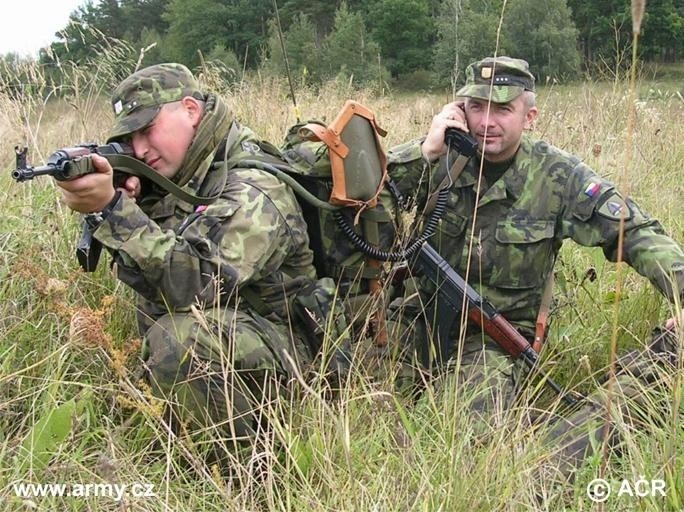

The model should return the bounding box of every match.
[282,117,402,343]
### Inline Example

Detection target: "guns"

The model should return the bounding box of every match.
[11,143,135,273]
[413,241,580,408]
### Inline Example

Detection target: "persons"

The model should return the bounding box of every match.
[360,54,683,440]
[51,60,350,483]
[536,310,684,484]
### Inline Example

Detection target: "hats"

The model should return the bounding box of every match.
[105,62,204,144]
[456,56,536,103]
